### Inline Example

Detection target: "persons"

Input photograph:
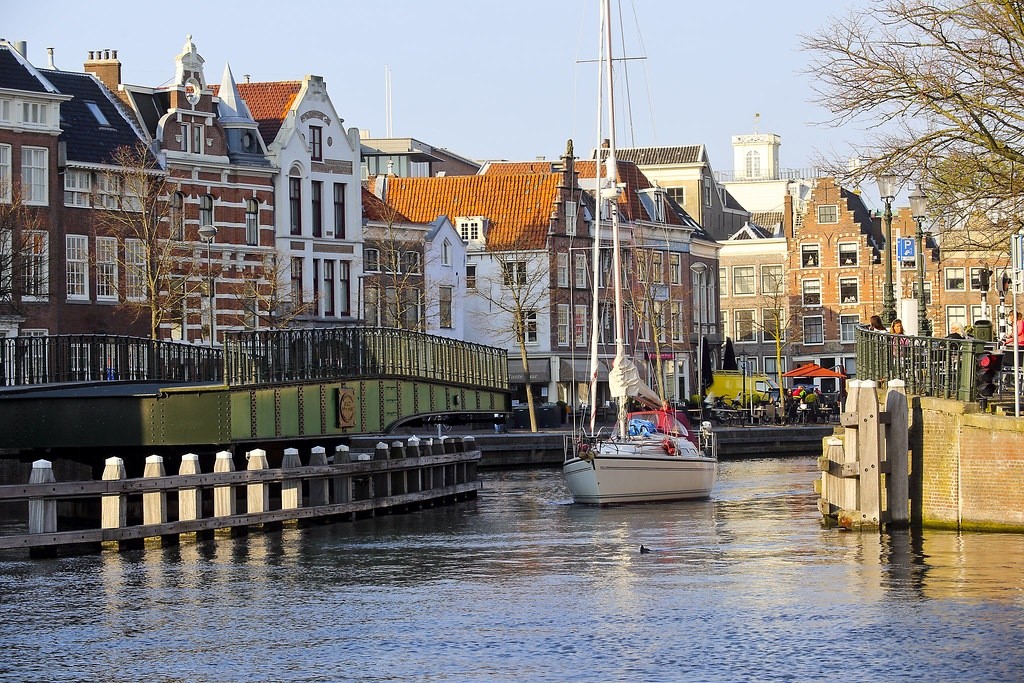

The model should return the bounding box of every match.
[659,402,674,433]
[868,316,887,333]
[784,384,840,424]
[1002,310,1024,385]
[891,319,909,356]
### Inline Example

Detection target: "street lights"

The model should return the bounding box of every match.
[704,368,780,408]
[198,223,218,347]
[907,182,932,335]
[690,261,707,410]
[875,164,896,324]
[354,272,375,327]
[740,347,749,422]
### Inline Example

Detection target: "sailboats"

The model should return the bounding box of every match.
[562,0,719,507]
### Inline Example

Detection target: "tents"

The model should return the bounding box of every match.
[781,360,847,380]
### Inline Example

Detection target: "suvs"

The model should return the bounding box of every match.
[761,384,826,408]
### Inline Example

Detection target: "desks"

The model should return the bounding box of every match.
[579,407,610,423]
[738,409,769,424]
[712,409,744,427]
[675,409,720,426]
[798,408,832,424]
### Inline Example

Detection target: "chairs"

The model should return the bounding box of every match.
[628,402,840,427]
[892,341,1024,398]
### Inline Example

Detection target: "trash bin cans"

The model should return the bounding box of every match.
[974,320,993,343]
[514,406,531,429]
[493,414,507,433]
[538,405,561,429]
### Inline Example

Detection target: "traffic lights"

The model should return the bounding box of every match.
[998,273,1011,294]
[978,263,994,292]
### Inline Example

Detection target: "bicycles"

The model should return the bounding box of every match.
[426,415,453,432]
[668,394,782,426]
